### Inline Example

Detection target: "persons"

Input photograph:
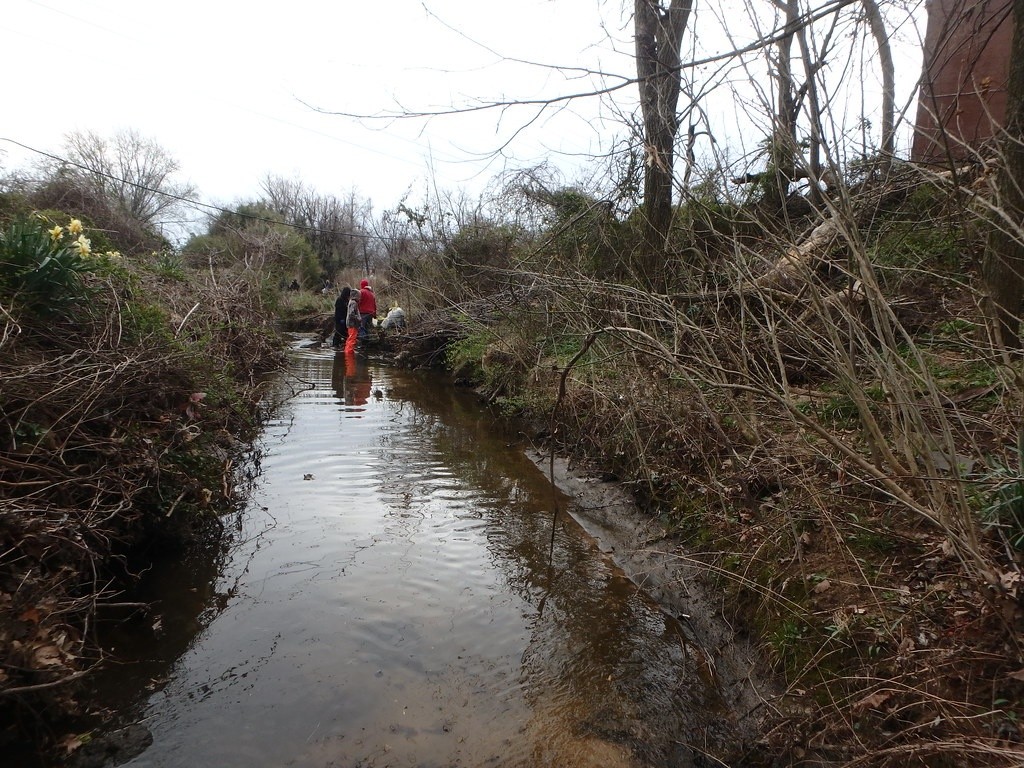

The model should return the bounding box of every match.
[333,287,351,346]
[344,289,363,353]
[359,279,377,336]
